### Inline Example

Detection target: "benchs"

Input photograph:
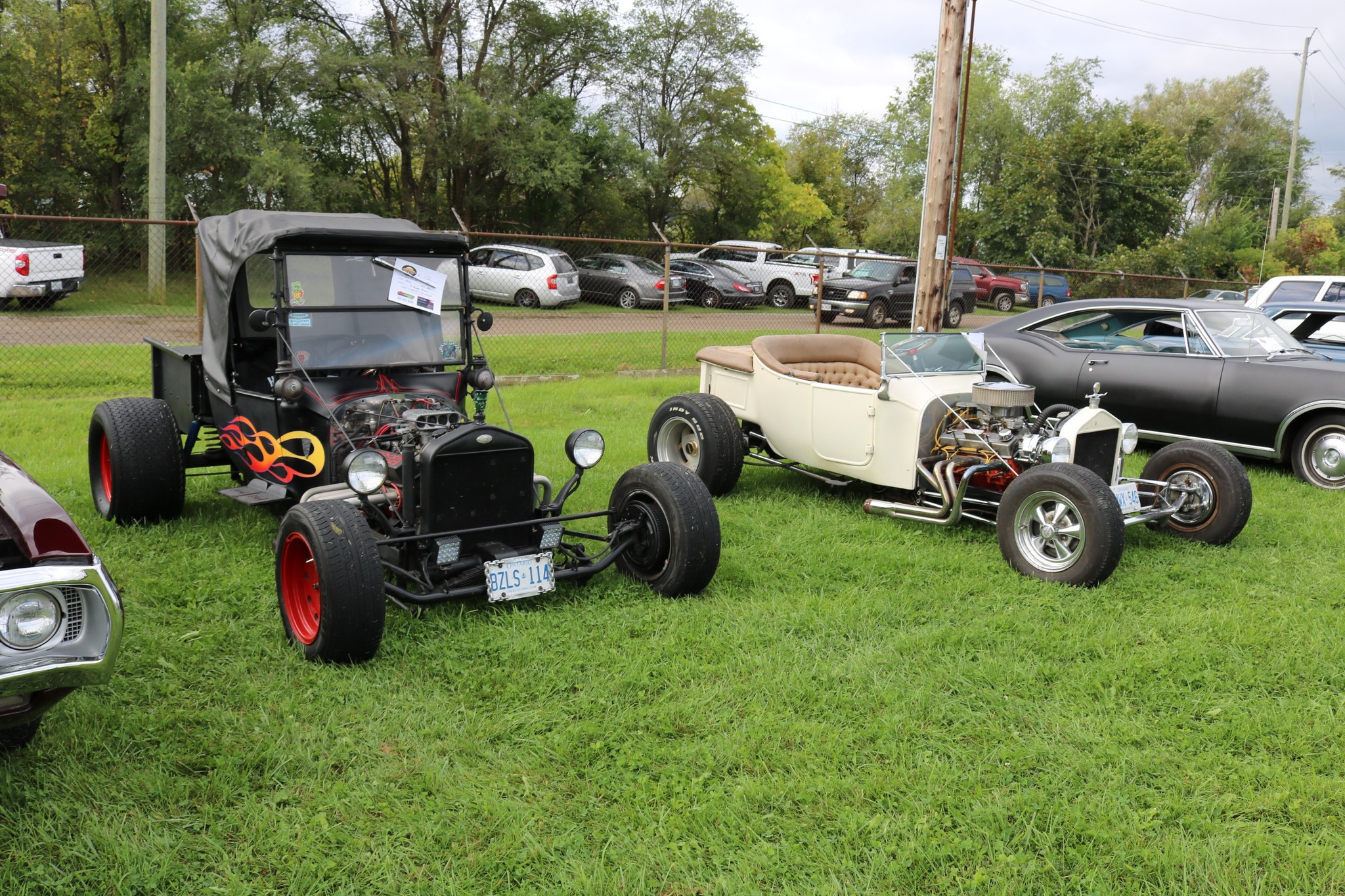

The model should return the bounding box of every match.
[238,334,408,394]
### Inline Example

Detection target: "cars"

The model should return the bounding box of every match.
[888,274,1344,490]
[571,254,687,310]
[0,456,125,749]
[656,260,766,310]
[645,331,1252,592]
[89,209,722,665]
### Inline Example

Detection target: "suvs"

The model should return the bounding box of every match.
[807,260,977,329]
[434,244,582,309]
[785,246,1071,311]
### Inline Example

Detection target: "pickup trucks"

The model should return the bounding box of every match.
[0,184,86,312]
[661,241,836,310]
